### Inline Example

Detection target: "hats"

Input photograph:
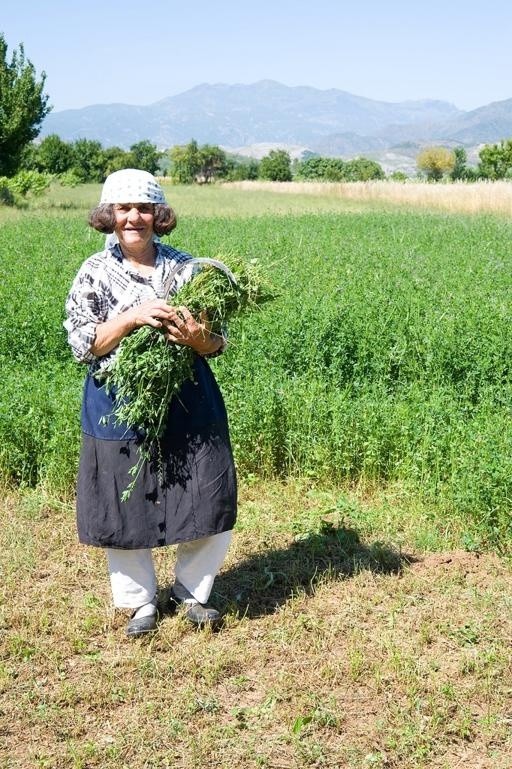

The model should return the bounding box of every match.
[100,168,167,205]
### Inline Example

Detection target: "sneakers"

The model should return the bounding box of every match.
[127,600,163,634]
[167,586,222,623]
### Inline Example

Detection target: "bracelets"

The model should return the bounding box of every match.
[206,348,223,360]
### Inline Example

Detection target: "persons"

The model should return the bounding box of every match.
[61,168,238,638]
